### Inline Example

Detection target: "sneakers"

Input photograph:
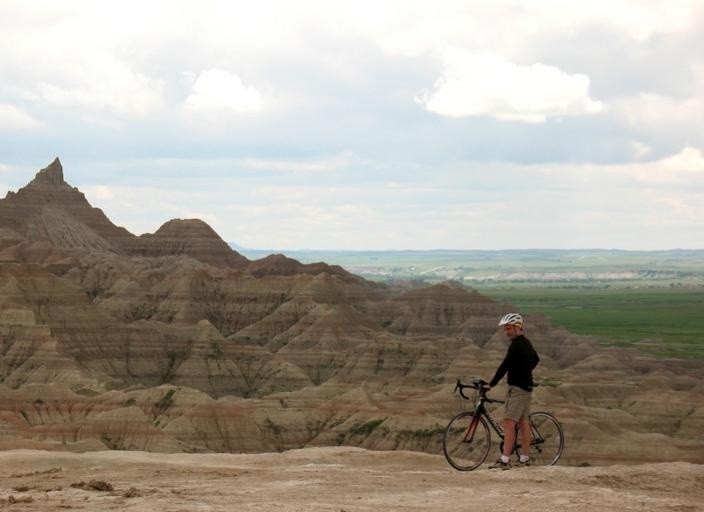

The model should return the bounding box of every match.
[488,458,512,469]
[513,458,530,466]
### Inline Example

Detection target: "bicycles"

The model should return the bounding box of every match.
[441,377,565,472]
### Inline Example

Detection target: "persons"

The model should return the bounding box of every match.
[480,312,540,470]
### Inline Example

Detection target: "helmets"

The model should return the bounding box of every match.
[497,312,523,328]
